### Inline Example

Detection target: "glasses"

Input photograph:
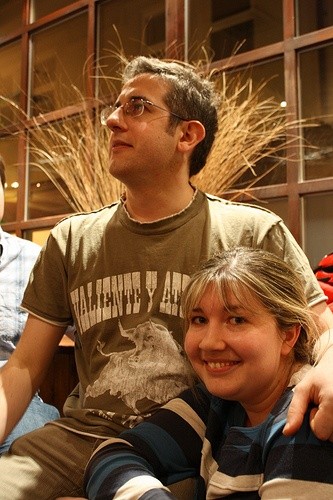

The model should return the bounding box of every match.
[100,98,189,126]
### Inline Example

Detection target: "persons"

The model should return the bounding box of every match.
[0,57,333,500]
[85,246,333,500]
